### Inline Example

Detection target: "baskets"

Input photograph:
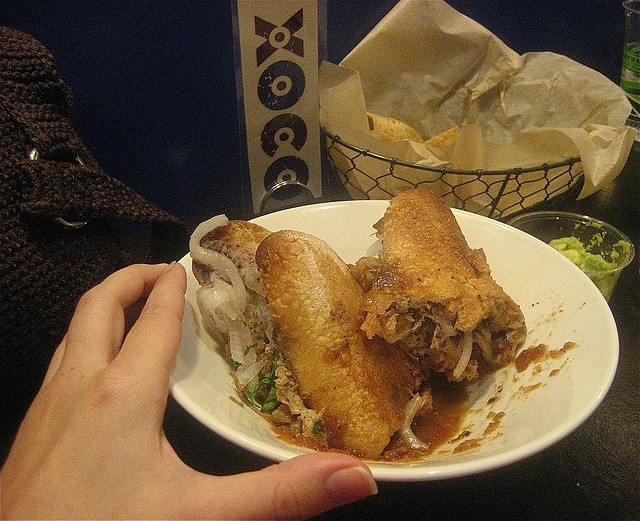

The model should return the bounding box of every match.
[320,125,638,219]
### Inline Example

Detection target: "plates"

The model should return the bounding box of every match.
[148,199,621,488]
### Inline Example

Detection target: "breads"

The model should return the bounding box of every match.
[192,221,274,289]
[254,230,423,462]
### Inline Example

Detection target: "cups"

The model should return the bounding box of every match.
[505,210,635,302]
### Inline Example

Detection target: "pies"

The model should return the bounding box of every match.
[353,191,527,380]
[365,113,423,142]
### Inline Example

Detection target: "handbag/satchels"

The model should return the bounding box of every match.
[1,29,187,395]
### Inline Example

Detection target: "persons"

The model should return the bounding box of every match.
[0,262,378,521]
